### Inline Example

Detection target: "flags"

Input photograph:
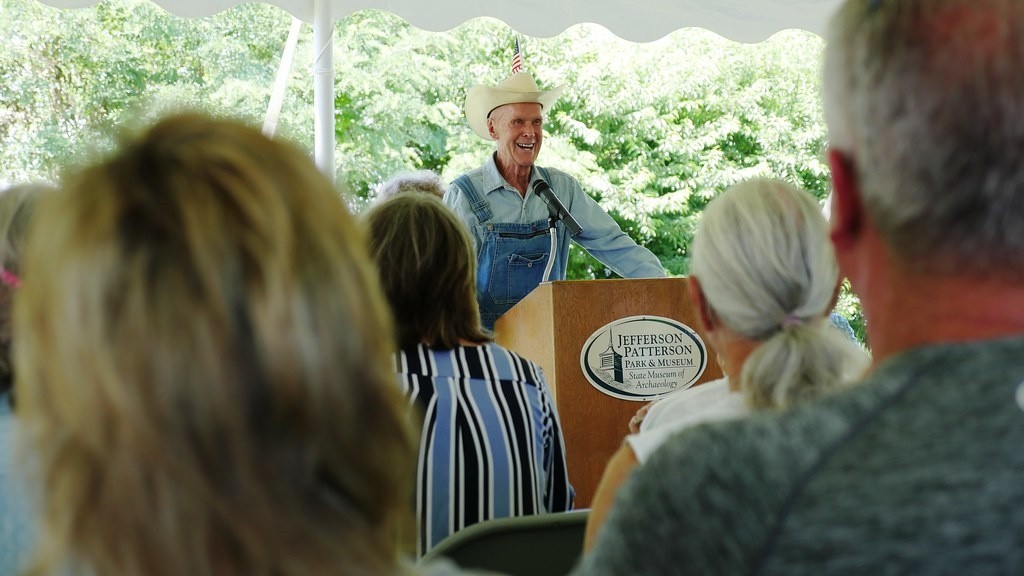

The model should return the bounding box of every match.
[511,36,522,74]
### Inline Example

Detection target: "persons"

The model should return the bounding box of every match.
[2,0,1023,576]
[442,71,684,340]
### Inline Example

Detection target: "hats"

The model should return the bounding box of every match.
[464,74,570,141]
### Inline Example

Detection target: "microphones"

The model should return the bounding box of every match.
[531,179,583,237]
